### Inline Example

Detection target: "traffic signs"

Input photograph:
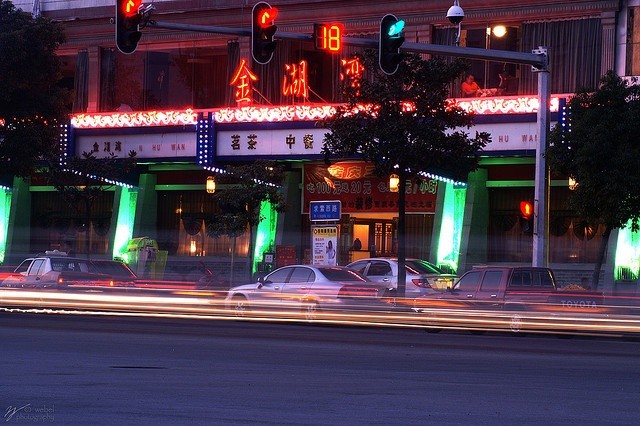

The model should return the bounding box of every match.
[308,200,343,222]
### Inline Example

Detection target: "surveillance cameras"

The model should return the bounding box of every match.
[446,5,465,25]
[140,4,155,23]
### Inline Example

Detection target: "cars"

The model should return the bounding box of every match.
[1,250,112,289]
[224,265,397,305]
[345,258,460,297]
[91,259,137,280]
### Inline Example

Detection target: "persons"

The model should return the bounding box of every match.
[460,75,480,94]
[326,241,335,259]
[498,73,507,88]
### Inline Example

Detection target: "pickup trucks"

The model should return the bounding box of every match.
[415,265,605,310]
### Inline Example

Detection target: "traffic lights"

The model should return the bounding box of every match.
[252,3,278,65]
[311,22,342,54]
[114,1,145,52]
[376,17,405,76]
[518,198,535,222]
